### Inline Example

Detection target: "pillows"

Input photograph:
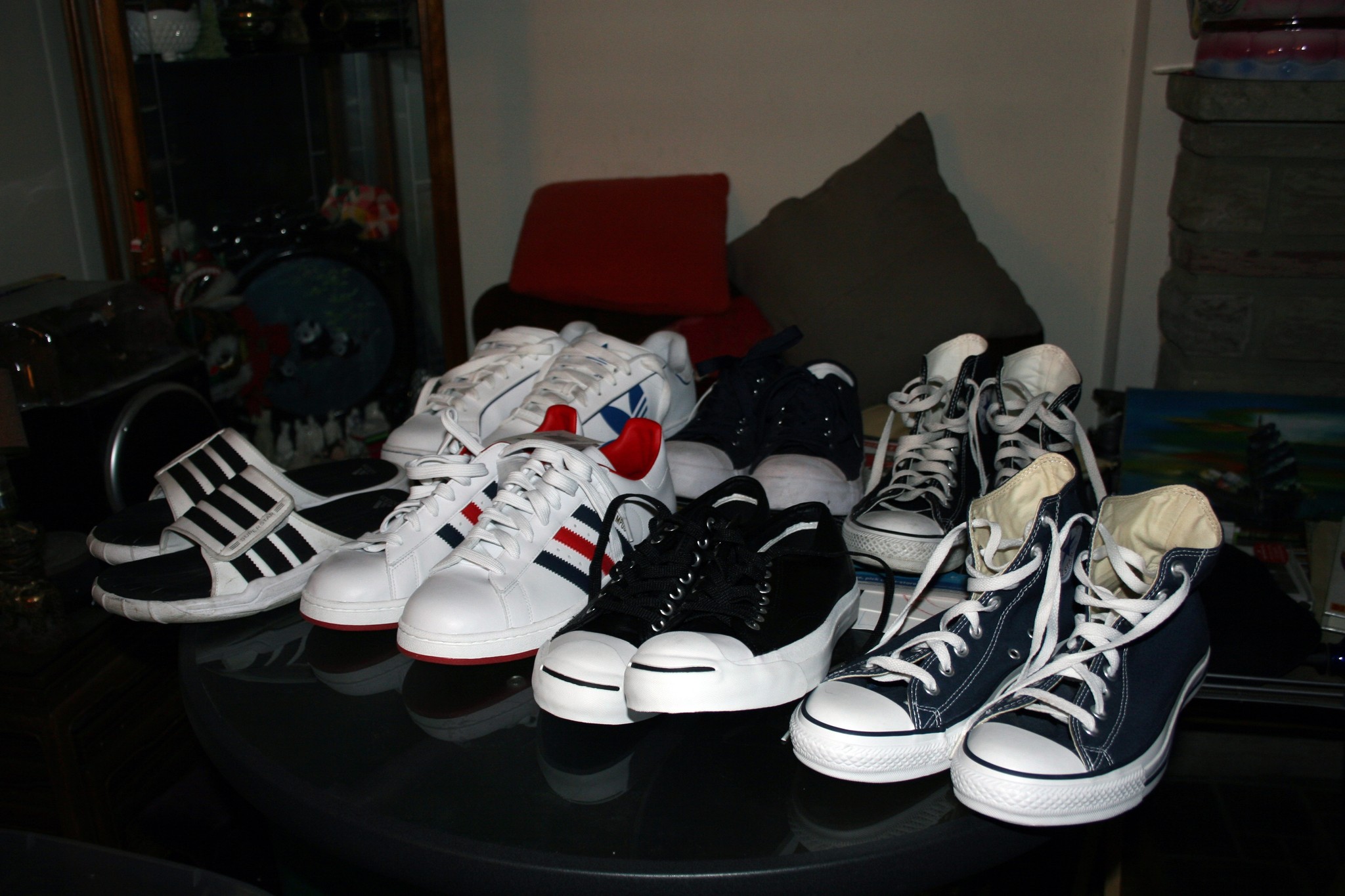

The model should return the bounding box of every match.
[508,112,1042,413]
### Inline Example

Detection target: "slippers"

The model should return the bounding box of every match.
[91,467,410,625]
[87,428,410,564]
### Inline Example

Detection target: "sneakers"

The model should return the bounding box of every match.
[842,333,989,574]
[484,327,715,454]
[791,452,1098,782]
[398,416,677,667]
[663,340,794,499]
[955,484,1223,828]
[298,404,582,630]
[381,322,596,467]
[751,361,868,515]
[965,342,1083,496]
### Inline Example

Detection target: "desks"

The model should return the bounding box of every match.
[177,458,1345,896]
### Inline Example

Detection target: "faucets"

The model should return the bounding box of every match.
[54,305,116,337]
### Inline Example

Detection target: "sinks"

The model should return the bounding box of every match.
[18,346,200,413]
